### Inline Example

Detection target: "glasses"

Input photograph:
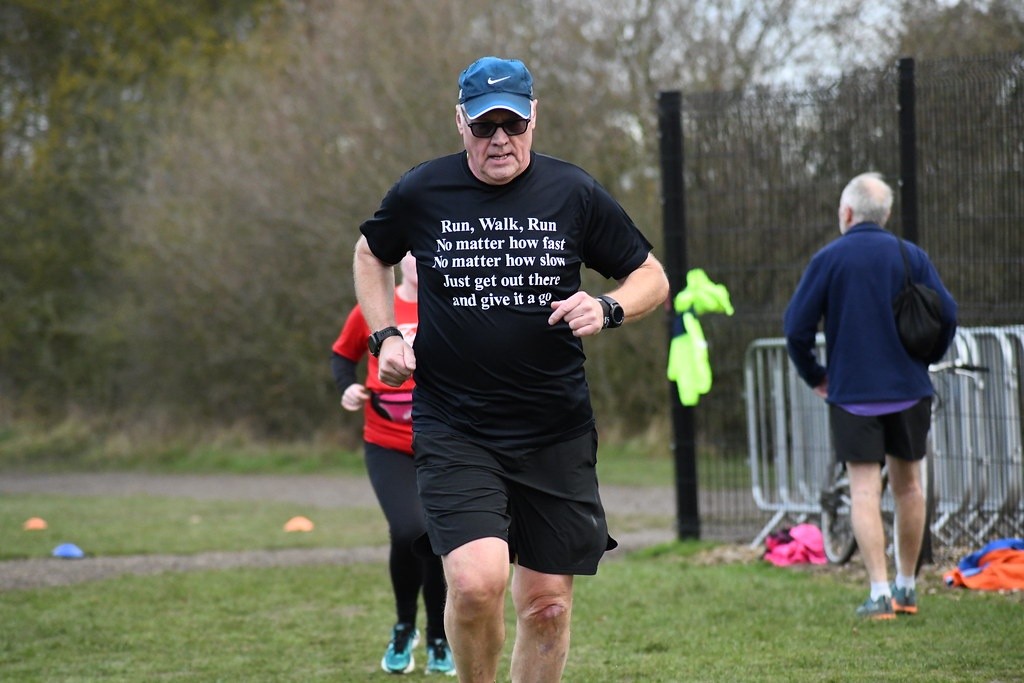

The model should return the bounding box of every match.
[460,107,531,138]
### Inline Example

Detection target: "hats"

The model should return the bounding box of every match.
[458,57,533,119]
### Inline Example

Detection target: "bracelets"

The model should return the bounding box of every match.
[595,297,612,330]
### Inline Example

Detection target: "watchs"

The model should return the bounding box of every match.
[368,324,402,357]
[598,295,624,329]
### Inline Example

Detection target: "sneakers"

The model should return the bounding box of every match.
[381,623,458,678]
[853,586,916,622]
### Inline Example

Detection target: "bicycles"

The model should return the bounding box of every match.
[817,359,990,579]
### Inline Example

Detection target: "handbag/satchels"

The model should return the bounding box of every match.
[893,239,944,362]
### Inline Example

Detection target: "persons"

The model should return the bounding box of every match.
[354,55,672,683]
[327,247,456,679]
[785,172,960,622]
